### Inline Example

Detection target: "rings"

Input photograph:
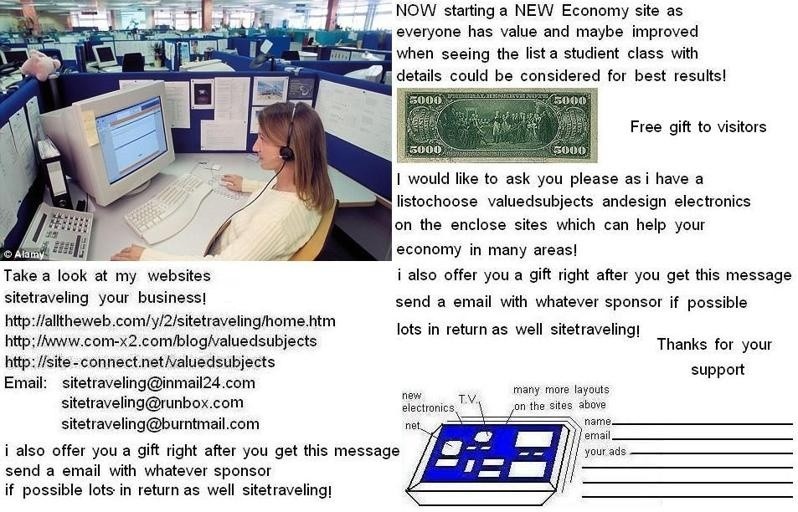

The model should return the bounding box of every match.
[233,176,237,180]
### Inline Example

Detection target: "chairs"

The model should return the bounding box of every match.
[289,198,337,260]
[281,51,300,60]
[123,52,144,71]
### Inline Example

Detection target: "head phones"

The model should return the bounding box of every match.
[279,103,297,162]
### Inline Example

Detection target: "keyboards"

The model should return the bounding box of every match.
[124,174,213,245]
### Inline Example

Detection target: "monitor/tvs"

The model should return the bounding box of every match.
[92,45,118,69]
[38,80,176,207]
[179,59,236,72]
[360,51,381,61]
[343,65,383,84]
[0,47,30,64]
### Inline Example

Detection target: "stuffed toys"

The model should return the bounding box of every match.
[18,47,60,82]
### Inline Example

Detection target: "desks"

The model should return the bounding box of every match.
[28,152,378,261]
[102,64,170,72]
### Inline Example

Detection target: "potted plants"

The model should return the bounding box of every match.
[151,41,164,68]
[15,15,42,37]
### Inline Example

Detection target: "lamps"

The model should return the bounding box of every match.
[249,53,276,71]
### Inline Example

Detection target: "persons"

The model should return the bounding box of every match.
[190,40,201,54]
[109,100,335,261]
[438,105,557,150]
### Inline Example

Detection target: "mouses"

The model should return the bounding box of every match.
[218,176,233,186]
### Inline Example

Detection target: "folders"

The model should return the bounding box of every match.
[35,135,74,210]
